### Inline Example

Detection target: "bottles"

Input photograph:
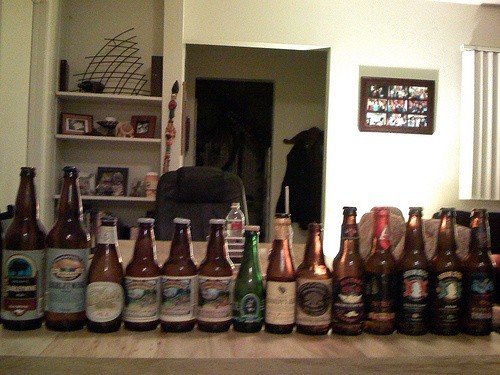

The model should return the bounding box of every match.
[86,218,124,330]
[226,201,244,239]
[364,207,397,336]
[43,167,91,331]
[332,207,363,336]
[296,223,332,336]
[124,217,157,332]
[231,225,264,332]
[265,213,296,334]
[146,171,159,197]
[158,218,197,332]
[0,166,46,332]
[197,218,234,333]
[397,206,430,334]
[461,208,495,336]
[430,207,463,335]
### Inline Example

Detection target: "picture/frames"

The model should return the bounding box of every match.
[359,76,435,135]
[60,112,94,135]
[97,167,128,196]
[130,115,157,138]
[77,172,96,196]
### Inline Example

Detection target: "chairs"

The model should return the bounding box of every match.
[156,166,249,242]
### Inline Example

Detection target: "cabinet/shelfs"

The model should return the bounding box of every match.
[52,90,163,203]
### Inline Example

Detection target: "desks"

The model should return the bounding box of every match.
[0,241,500,375]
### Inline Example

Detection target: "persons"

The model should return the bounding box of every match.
[365,84,428,127]
